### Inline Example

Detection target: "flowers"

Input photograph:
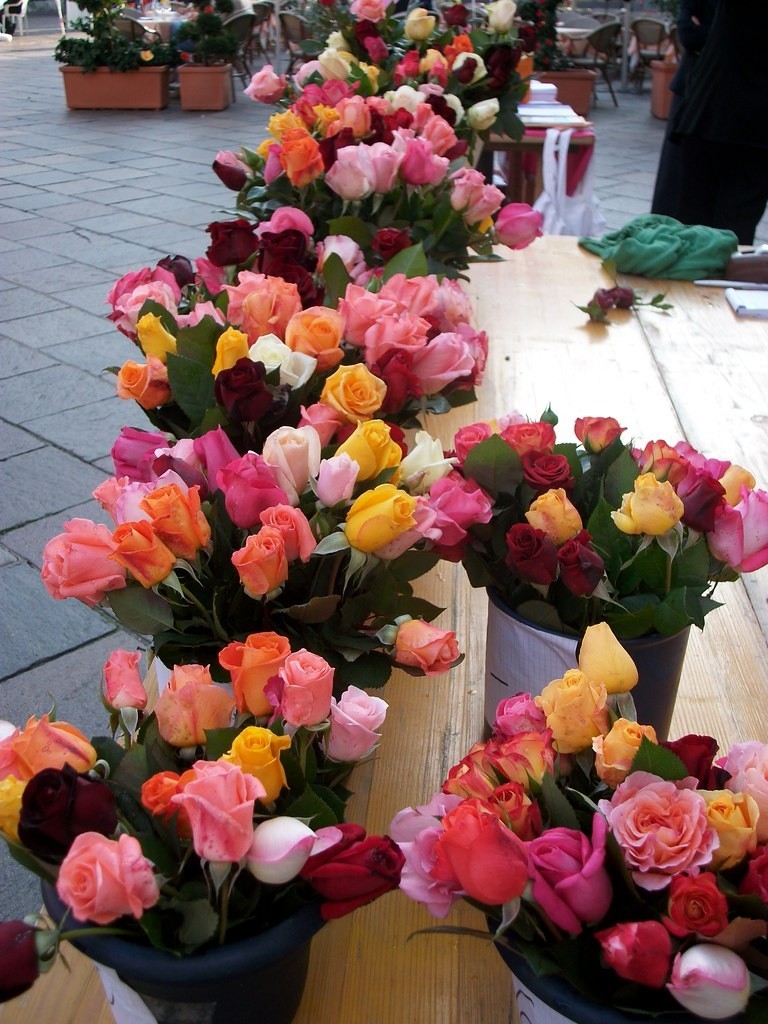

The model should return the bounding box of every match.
[36,77,547,700]
[53,0,188,75]
[244,0,573,77]
[425,399,766,640]
[386,620,768,1024]
[1,630,408,1007]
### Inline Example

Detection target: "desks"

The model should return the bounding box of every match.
[0,231,768,1024]
[469,95,597,209]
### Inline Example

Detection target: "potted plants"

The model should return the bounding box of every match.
[172,13,243,113]
[646,0,686,121]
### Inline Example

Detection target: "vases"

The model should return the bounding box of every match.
[480,585,696,745]
[479,913,635,1024]
[530,66,597,117]
[58,64,172,112]
[37,874,332,1024]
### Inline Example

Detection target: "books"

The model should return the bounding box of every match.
[724,288,767,317]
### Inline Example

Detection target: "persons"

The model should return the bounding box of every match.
[169,0,256,93]
[0,0,23,42]
[649,0,768,246]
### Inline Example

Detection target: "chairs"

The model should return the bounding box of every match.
[2,0,29,36]
[548,5,683,108]
[104,0,329,107]
